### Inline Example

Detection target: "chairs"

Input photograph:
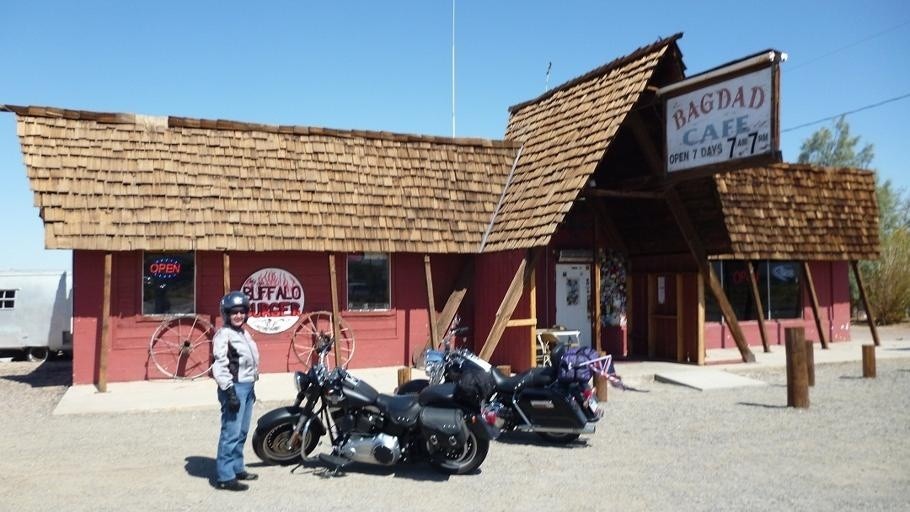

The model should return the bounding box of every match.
[541,333,559,351]
[537,332,554,368]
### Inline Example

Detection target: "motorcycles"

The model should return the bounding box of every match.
[396,314,619,443]
[252,330,504,475]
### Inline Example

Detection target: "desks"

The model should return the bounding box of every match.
[536,329,580,352]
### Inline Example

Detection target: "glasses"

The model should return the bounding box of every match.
[227,310,246,314]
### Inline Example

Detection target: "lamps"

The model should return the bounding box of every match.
[590,178,597,189]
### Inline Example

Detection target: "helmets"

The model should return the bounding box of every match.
[221,291,249,323]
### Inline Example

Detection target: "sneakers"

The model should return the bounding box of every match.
[236,472,258,480]
[219,480,248,490]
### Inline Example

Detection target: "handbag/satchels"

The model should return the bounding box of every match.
[453,368,488,405]
[557,346,599,385]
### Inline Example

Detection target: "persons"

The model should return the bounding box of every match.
[211,291,260,492]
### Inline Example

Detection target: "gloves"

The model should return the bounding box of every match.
[226,384,240,412]
